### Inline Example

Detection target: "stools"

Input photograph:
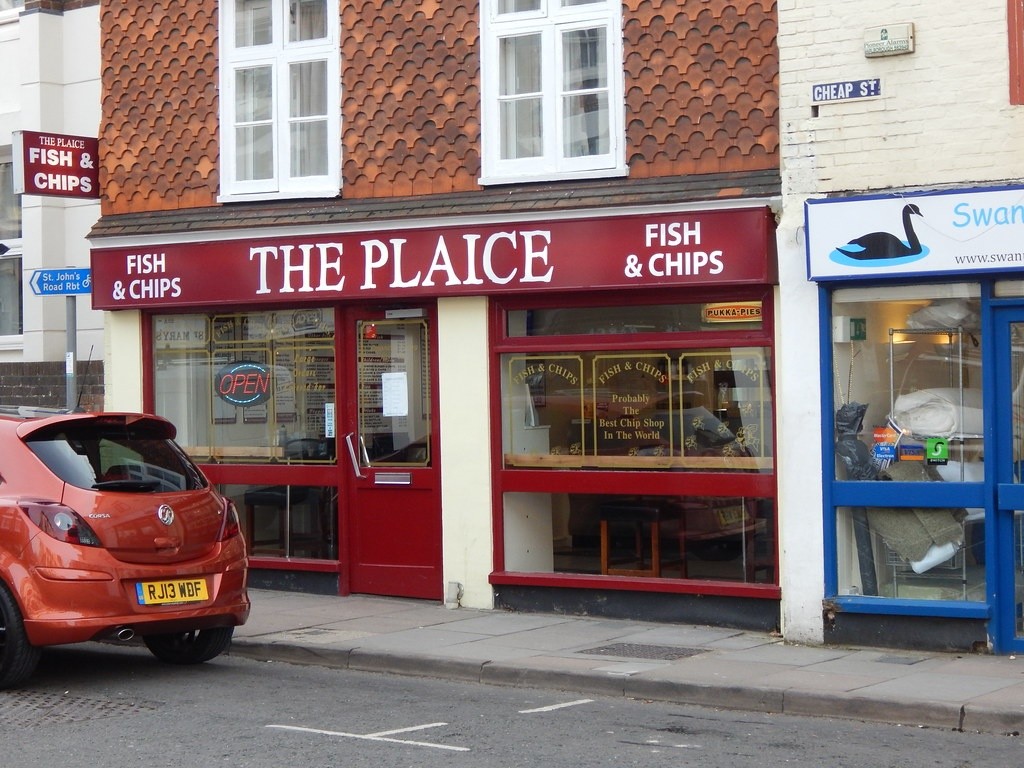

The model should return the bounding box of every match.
[598,502,687,582]
[245,484,314,556]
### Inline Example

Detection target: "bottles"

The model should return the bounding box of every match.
[278,423,287,446]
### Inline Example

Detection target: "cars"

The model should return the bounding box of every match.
[0,405,251,690]
[368,387,761,567]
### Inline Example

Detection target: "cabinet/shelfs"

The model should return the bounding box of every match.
[887,326,1024,601]
